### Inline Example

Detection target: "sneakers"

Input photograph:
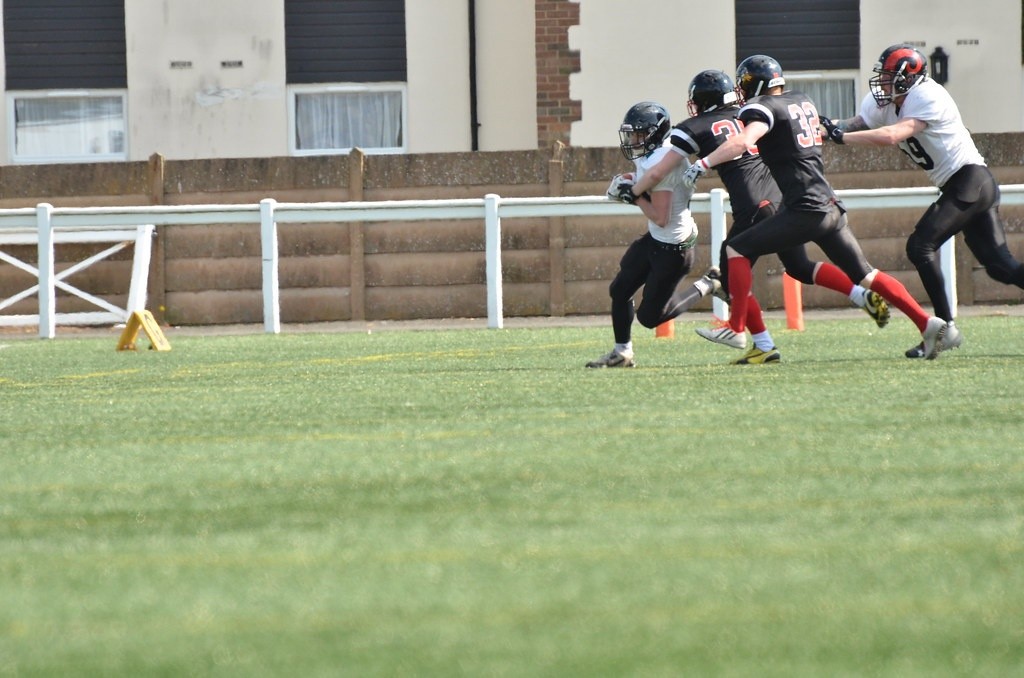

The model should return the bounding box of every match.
[585,348,636,369]
[860,287,890,329]
[703,266,730,307]
[905,320,962,359]
[695,327,747,349]
[920,316,947,361]
[729,341,781,365]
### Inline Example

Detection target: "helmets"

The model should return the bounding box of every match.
[620,102,670,132]
[734,55,784,88]
[872,44,928,75]
[688,69,734,94]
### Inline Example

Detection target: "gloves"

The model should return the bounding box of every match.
[605,174,634,202]
[617,183,640,204]
[681,157,711,191]
[818,116,845,145]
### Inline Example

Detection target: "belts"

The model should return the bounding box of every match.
[661,244,682,251]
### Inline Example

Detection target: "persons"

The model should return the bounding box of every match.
[608,69,890,363]
[820,43,1024,357]
[682,55,950,361]
[585,102,729,370]
